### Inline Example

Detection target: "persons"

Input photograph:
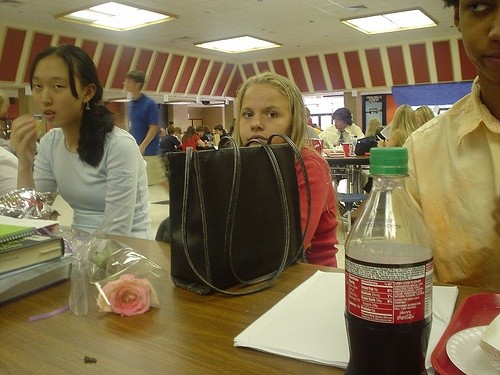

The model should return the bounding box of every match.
[124,70,160,185]
[365,116,381,137]
[378,104,419,147]
[233,71,340,268]
[401,0,500,292]
[0,88,17,196]
[415,106,435,127]
[228,118,236,136]
[304,106,373,193]
[10,44,152,240]
[159,117,230,155]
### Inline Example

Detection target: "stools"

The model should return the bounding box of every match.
[333,193,366,235]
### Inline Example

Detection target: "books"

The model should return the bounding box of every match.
[0,224,73,302]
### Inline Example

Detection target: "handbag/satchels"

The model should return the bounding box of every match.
[169,133,311,294]
[355,136,378,155]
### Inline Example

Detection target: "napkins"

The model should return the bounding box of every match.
[234,270,459,375]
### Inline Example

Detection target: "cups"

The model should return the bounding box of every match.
[313,138,324,153]
[342,143,353,158]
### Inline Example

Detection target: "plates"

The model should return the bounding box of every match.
[446,326,500,375]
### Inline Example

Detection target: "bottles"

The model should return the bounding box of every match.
[343,148,434,375]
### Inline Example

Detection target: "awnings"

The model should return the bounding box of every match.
[391,80,473,106]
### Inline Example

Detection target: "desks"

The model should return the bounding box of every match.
[324,154,369,193]
[0,235,500,375]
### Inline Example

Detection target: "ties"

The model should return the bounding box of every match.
[339,130,344,145]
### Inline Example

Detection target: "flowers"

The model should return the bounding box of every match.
[96,274,152,317]
